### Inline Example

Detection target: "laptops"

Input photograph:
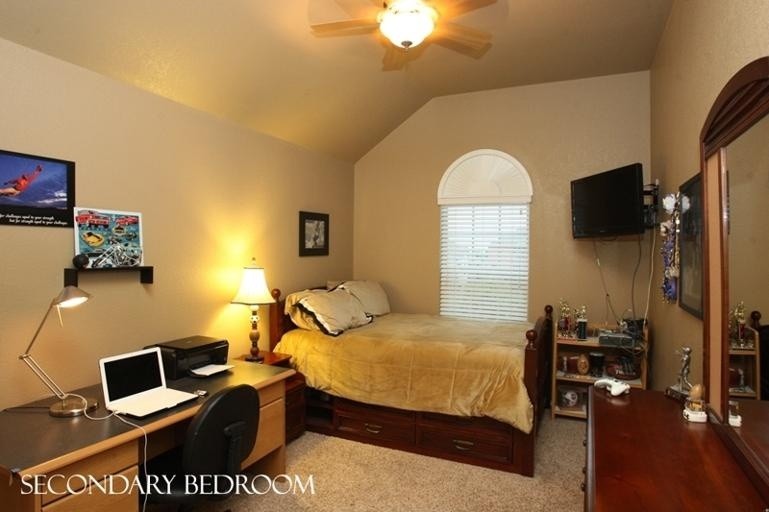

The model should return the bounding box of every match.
[99,345,199,420]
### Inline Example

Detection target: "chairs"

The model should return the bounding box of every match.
[152,384,261,510]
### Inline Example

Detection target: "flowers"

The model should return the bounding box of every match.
[658,192,679,305]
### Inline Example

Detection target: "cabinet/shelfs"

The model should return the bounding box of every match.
[550,309,650,421]
[726,326,762,401]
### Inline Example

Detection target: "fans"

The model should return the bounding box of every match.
[308,0,495,76]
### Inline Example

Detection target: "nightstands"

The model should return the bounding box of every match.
[235,352,307,444]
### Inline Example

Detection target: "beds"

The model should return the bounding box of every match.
[751,311,768,402]
[270,280,553,477]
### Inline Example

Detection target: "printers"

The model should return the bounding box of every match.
[143,335,229,380]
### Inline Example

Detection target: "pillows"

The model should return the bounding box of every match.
[281,289,323,331]
[331,278,391,319]
[301,285,372,336]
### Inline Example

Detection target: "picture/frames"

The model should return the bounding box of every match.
[73,210,145,270]
[298,210,331,257]
[0,149,75,229]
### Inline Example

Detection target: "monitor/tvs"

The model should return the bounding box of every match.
[570,163,660,241]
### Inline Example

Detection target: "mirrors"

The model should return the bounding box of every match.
[677,172,703,320]
[720,111,768,480]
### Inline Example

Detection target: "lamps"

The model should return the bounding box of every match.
[374,2,439,51]
[20,267,92,420]
[231,268,277,364]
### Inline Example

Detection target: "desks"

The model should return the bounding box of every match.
[0,349,298,511]
[589,377,765,511]
